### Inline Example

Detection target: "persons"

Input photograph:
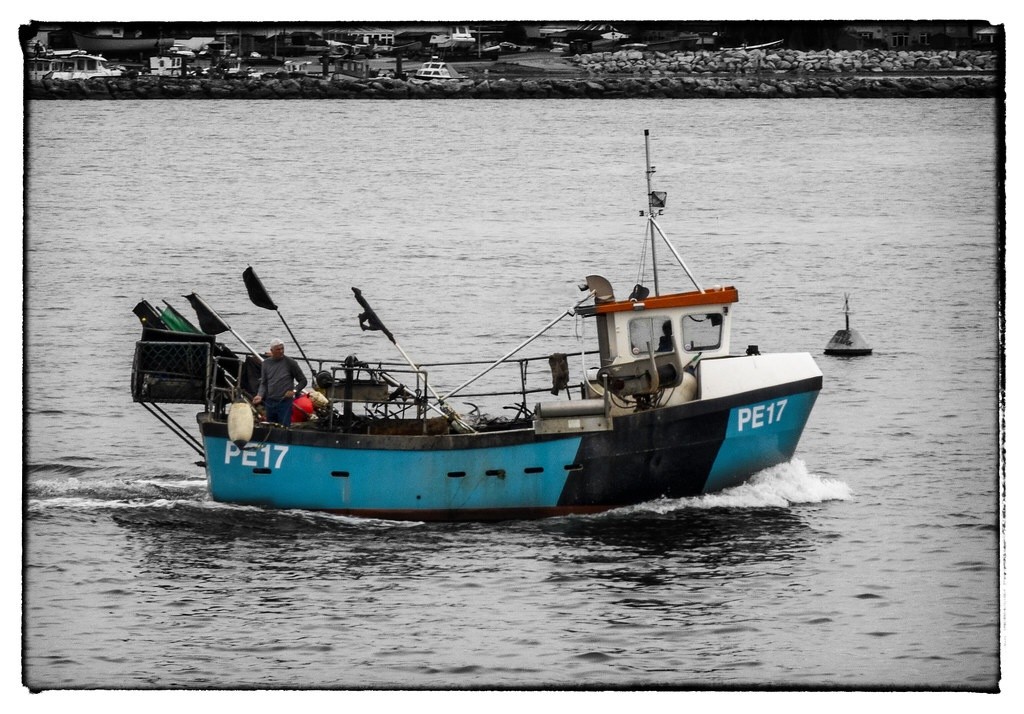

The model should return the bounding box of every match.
[656,319,675,351]
[253,338,306,428]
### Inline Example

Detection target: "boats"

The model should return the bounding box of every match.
[717,38,784,51]
[411,54,471,85]
[129,126,825,525]
[821,291,875,354]
